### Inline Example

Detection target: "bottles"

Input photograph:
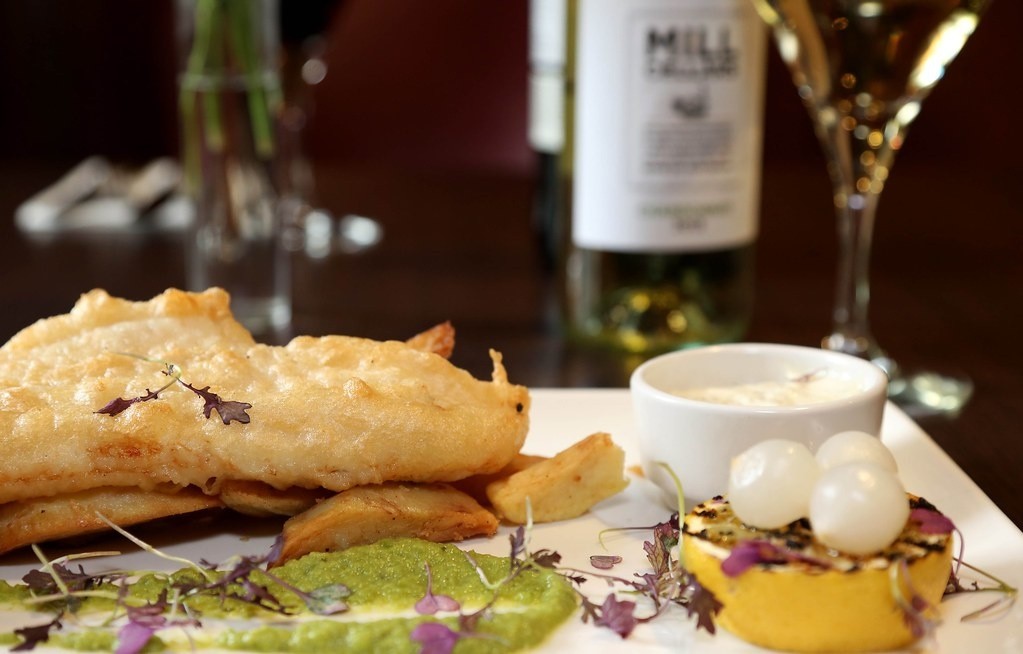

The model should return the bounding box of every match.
[522,0,767,353]
[179,0,295,339]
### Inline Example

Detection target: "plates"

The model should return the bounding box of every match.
[0,389,1022,654]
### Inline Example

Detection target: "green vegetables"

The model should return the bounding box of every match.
[7,459,1021,653]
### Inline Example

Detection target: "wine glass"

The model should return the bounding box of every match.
[753,0,988,401]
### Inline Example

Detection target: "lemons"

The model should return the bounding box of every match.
[683,495,952,653]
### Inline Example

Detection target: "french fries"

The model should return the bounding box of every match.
[0,434,631,574]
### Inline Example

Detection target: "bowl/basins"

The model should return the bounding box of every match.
[630,342,889,515]
